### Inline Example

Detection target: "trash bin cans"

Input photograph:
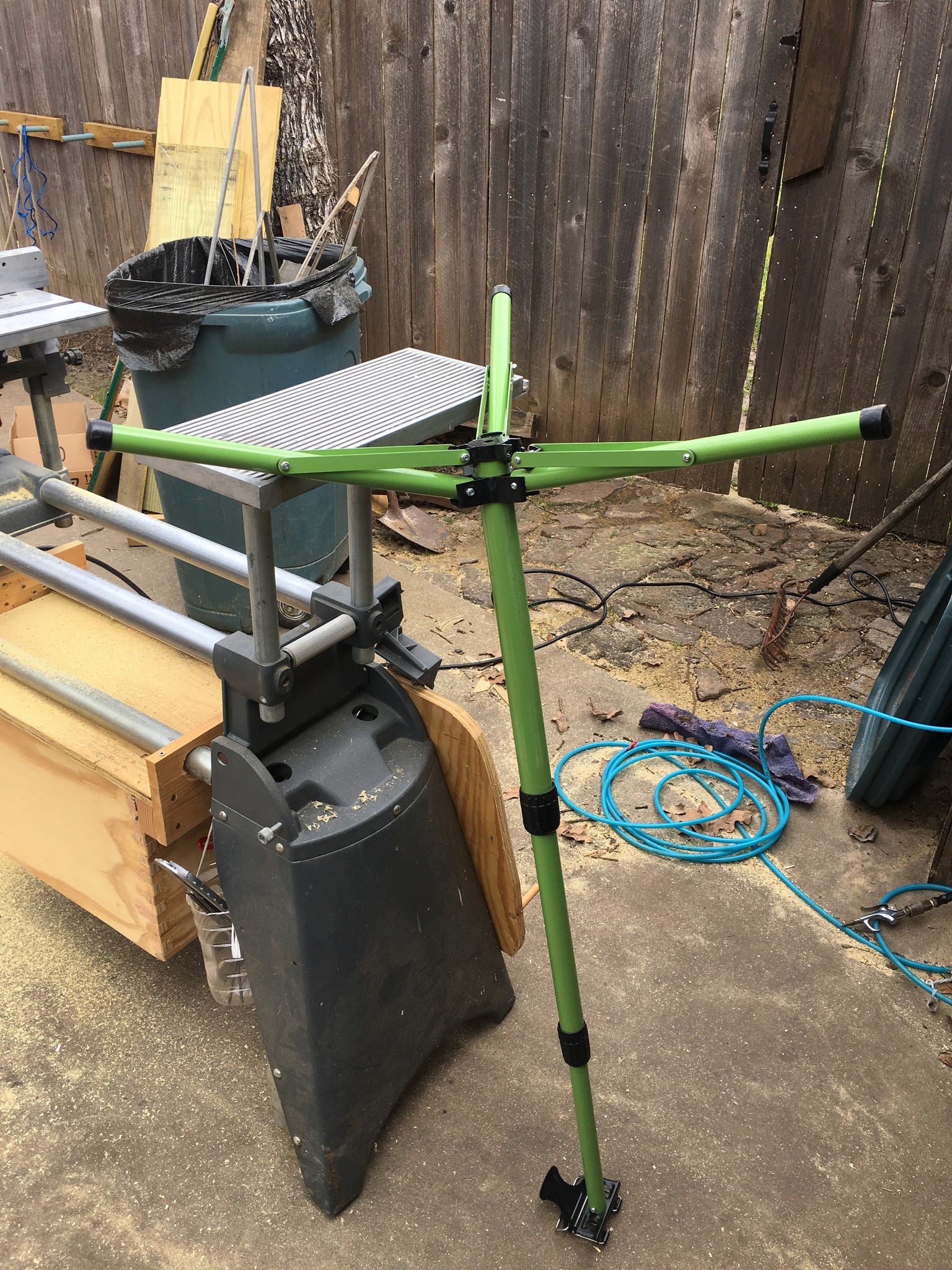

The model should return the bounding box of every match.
[102,236,372,633]
